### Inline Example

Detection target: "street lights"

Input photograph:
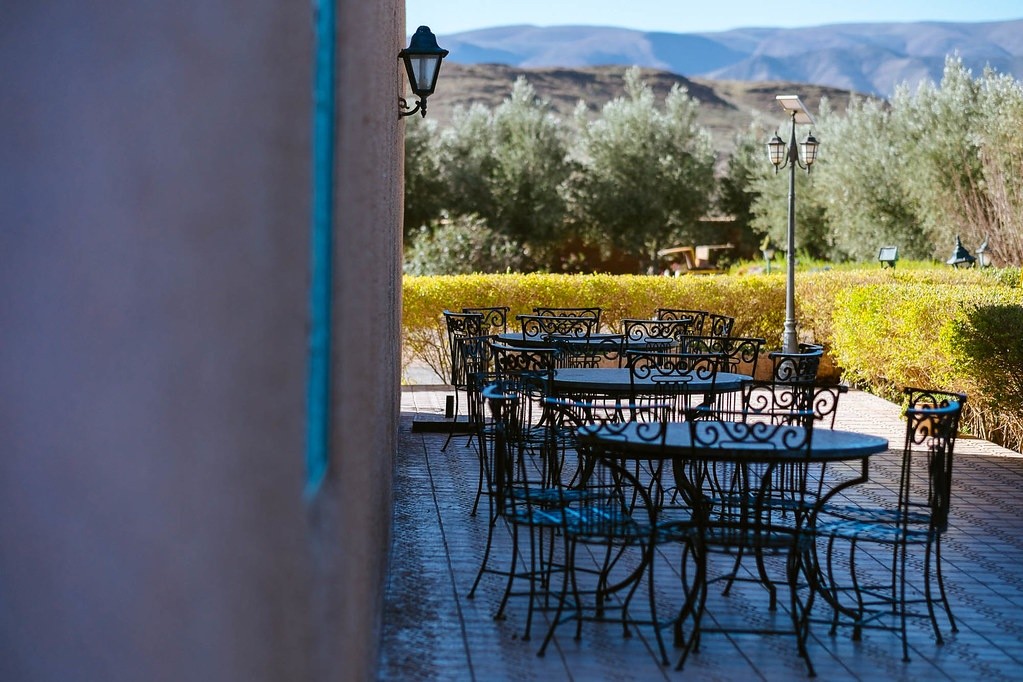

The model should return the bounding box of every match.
[764,95,823,391]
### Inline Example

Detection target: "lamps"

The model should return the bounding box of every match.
[947,237,976,270]
[978,236,994,267]
[397,21,449,120]
[879,245,900,262]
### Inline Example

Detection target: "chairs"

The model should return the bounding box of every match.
[445,305,965,668]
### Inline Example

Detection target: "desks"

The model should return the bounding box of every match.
[493,332,680,479]
[579,425,889,676]
[534,365,760,536]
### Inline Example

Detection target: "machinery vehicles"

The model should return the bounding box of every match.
[655,242,737,276]
